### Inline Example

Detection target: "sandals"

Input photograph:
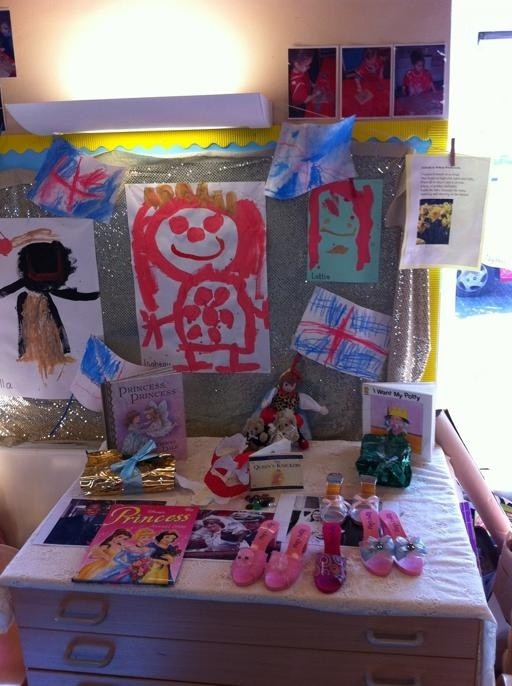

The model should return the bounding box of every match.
[231,471,427,593]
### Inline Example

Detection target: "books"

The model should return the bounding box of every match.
[71,505,200,587]
[362,379,438,466]
[98,364,189,463]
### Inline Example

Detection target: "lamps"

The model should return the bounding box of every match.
[5,92,276,135]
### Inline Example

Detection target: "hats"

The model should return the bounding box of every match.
[385,407,410,424]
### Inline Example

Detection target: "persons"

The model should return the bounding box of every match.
[44,499,106,546]
[183,518,249,560]
[289,47,436,110]
[287,503,364,547]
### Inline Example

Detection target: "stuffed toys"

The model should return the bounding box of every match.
[238,354,329,450]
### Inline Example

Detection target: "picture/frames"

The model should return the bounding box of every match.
[340,43,393,119]
[393,41,448,119]
[286,45,339,120]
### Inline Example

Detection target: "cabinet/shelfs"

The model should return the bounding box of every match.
[0,439,498,686]
